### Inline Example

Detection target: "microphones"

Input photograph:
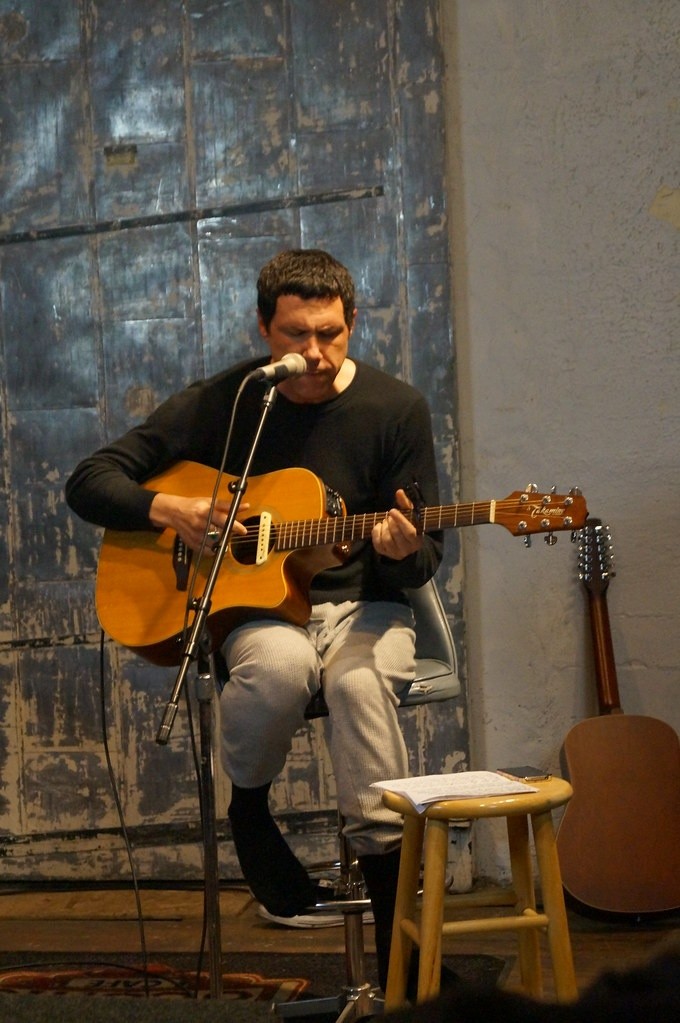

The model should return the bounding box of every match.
[248,353,307,380]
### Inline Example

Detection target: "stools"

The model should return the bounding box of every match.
[381,776,577,1011]
[203,573,463,1023]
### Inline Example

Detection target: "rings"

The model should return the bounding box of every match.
[206,526,220,543]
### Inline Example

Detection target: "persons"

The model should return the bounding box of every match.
[63,245,446,1002]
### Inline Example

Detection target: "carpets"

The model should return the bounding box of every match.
[0,949,517,1023]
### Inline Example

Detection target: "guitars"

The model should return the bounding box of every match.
[553,515,680,929]
[93,459,591,670]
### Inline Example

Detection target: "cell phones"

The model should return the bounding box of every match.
[497,764,550,782]
